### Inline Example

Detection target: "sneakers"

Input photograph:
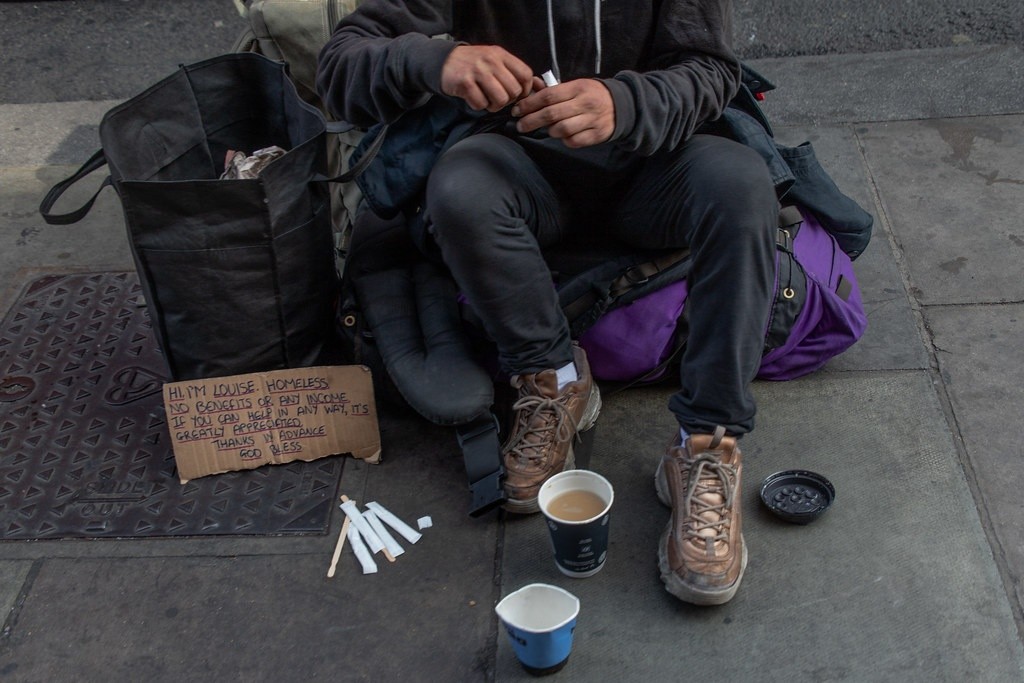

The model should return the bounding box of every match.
[501,340,602,513]
[655,417,748,605]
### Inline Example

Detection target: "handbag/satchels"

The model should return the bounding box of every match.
[40,54,389,381]
[579,211,867,381]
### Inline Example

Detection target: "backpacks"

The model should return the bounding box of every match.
[229,0,368,259]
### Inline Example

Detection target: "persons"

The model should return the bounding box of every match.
[315,1,778,607]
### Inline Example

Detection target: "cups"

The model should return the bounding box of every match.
[537,470,615,579]
[495,582,580,677]
